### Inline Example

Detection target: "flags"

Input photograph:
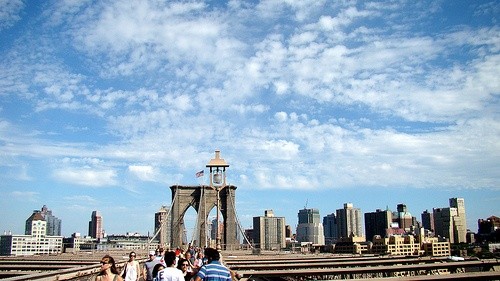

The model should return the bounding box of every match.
[195,170,204,178]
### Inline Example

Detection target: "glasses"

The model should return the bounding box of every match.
[100,261,109,266]
[130,255,136,257]
[182,263,189,266]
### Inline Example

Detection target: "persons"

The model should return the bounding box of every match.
[142,246,208,281]
[96,254,123,281]
[195,249,233,281]
[155,250,185,281]
[120,251,140,281]
